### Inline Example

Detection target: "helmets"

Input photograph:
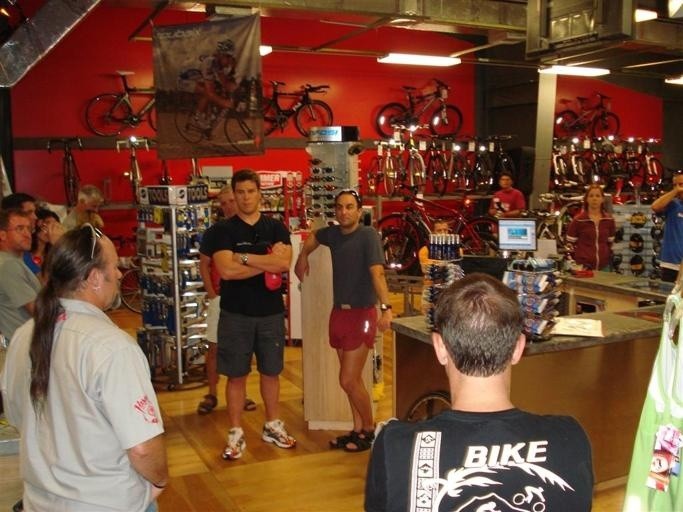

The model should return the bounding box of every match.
[218,39,234,57]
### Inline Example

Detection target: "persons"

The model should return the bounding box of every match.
[650,170,682,282]
[197,186,256,415]
[188,38,239,130]
[212,168,296,460]
[1,223,168,511]
[294,189,393,452]
[419,220,464,272]
[566,186,617,271]
[0,192,105,355]
[364,273,594,512]
[488,170,526,218]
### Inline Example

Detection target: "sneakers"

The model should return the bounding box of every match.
[223,428,246,461]
[261,420,296,448]
[191,116,212,131]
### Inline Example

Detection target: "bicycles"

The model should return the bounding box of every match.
[378,183,515,275]
[375,80,461,141]
[45,135,82,205]
[146,138,172,185]
[84,70,155,137]
[552,136,664,190]
[522,192,641,260]
[175,77,262,157]
[553,92,619,143]
[367,122,516,197]
[263,77,334,137]
[118,227,141,315]
[188,158,202,177]
[111,137,150,203]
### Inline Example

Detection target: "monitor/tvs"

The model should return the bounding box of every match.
[496,218,539,251]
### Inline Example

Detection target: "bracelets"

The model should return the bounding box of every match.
[242,252,249,266]
[378,304,392,311]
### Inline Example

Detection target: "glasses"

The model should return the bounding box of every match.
[672,169,683,175]
[337,189,361,203]
[80,222,101,257]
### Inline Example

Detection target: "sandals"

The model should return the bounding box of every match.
[244,399,256,411]
[198,395,217,413]
[331,428,374,452]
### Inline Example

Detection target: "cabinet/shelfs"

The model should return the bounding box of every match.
[555,135,670,212]
[136,185,212,386]
[1,137,508,287]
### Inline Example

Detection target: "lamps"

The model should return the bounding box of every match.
[664,74,683,85]
[376,53,461,67]
[536,66,611,77]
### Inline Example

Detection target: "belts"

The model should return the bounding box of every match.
[332,303,363,311]
